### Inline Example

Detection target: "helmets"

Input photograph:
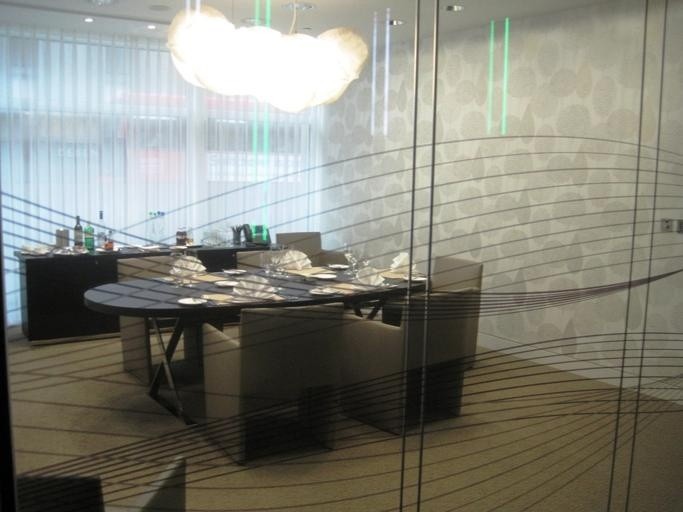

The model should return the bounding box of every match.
[178,264,427,306]
[53,242,202,256]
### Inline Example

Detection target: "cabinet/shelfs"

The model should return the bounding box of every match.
[74,211,113,250]
[176,227,194,245]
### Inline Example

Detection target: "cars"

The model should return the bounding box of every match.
[56,229,69,246]
[233,231,241,245]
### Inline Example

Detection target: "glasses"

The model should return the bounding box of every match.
[164,1,371,116]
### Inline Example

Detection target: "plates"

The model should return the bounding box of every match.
[12,242,281,349]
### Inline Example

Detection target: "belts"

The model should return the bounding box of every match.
[344,244,369,280]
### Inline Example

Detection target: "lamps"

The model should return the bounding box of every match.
[234,248,289,271]
[275,231,347,268]
[345,285,483,438]
[381,255,484,324]
[197,301,346,468]
[115,254,175,387]
